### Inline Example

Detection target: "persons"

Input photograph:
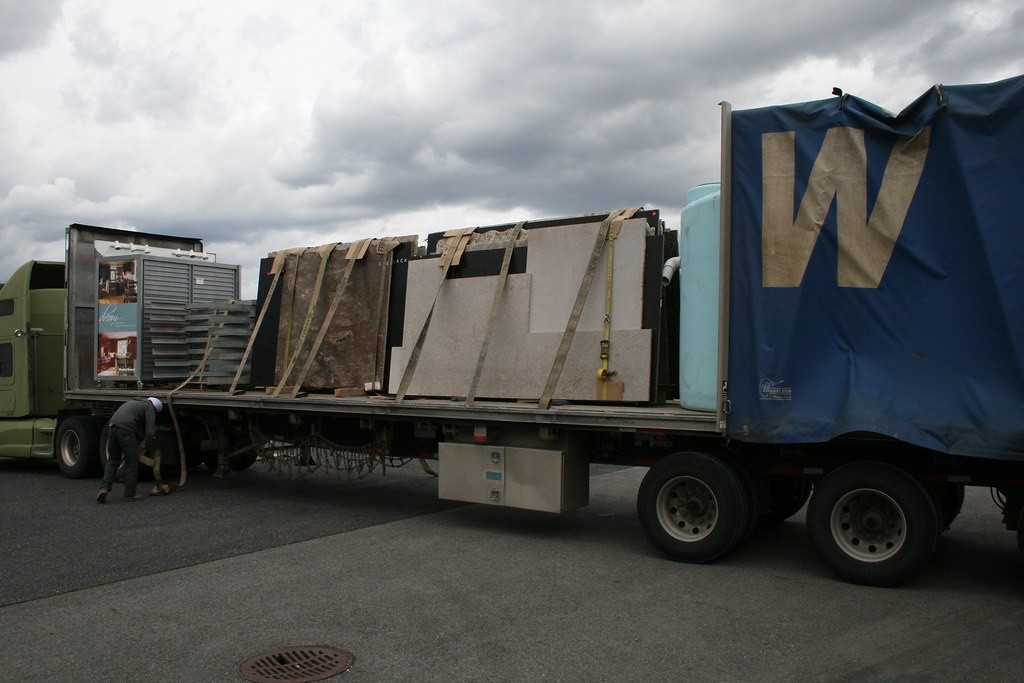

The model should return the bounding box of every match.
[96,397,163,502]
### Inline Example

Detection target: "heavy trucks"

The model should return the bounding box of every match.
[1,75,1023,586]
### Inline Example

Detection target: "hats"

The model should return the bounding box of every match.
[148,397,163,412]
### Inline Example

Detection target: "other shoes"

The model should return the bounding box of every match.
[96,487,107,501]
[125,494,144,501]
